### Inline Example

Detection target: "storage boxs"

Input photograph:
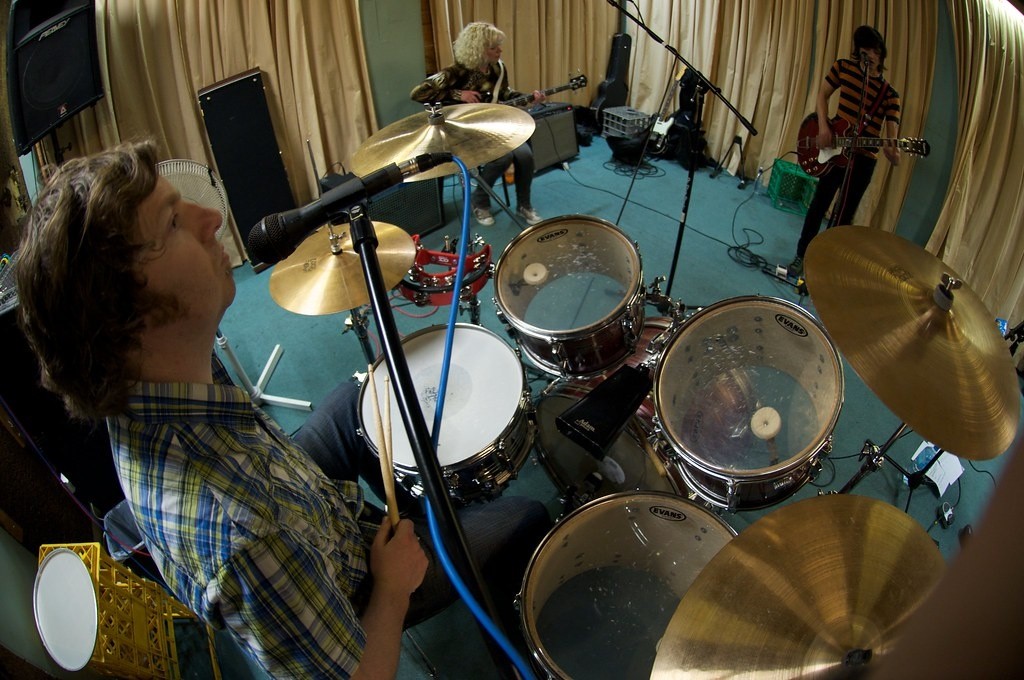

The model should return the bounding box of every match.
[766,157,820,216]
[601,107,651,140]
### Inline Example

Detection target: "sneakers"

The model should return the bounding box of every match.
[517,205,543,225]
[471,202,495,226]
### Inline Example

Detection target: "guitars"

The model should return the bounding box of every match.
[479,69,588,107]
[796,111,931,178]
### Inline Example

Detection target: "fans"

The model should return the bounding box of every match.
[152,158,312,424]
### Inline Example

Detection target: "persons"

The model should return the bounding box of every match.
[788,25,902,276]
[410,22,547,225]
[18,141,427,679]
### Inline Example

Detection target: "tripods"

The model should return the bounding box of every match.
[885,450,944,514]
[761,64,869,307]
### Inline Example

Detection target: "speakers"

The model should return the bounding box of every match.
[525,102,580,173]
[366,175,445,238]
[6,0,105,157]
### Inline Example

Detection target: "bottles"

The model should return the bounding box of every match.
[898,445,939,485]
[753,166,763,194]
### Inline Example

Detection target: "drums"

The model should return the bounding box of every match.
[534,316,716,507]
[653,295,845,512]
[493,214,647,380]
[514,489,739,680]
[356,322,536,506]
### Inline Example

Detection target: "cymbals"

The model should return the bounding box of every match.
[269,221,417,316]
[648,494,946,680]
[804,225,1020,461]
[349,103,536,183]
[396,234,491,307]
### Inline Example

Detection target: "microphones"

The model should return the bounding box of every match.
[860,50,869,66]
[246,152,453,266]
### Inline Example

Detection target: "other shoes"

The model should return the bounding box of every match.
[787,260,804,277]
